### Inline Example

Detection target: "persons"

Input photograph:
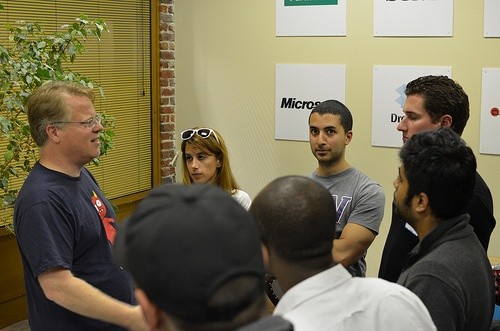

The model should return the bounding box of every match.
[266,99,386,314]
[392,127,496,331]
[12,79,155,331]
[245,175,438,331]
[378,75,497,284]
[180,127,252,212]
[110,183,294,331]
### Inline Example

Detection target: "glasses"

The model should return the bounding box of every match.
[181,127,220,146]
[50,114,98,129]
